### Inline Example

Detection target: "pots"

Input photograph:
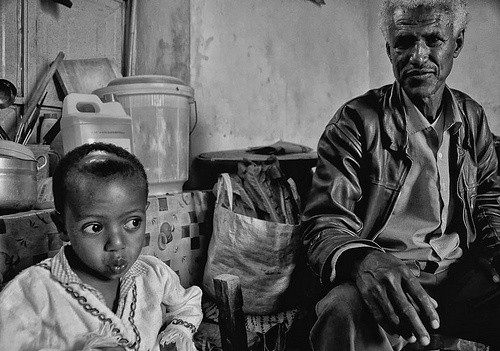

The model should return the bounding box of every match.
[0,155,47,213]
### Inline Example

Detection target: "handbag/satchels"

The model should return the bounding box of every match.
[203,172,303,314]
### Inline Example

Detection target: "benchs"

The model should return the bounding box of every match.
[0,189,307,350]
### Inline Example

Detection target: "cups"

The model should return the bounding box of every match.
[27,143,50,186]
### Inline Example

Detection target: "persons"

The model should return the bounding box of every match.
[0,142,203,351]
[294,0,500,351]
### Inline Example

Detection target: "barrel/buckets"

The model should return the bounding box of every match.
[59,93,132,153]
[91,75,198,198]
